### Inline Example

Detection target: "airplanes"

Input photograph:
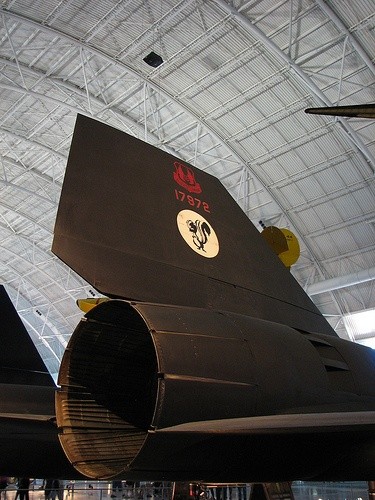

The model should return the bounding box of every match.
[0,112,375,486]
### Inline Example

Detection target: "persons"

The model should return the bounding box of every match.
[0,477,174,500]
